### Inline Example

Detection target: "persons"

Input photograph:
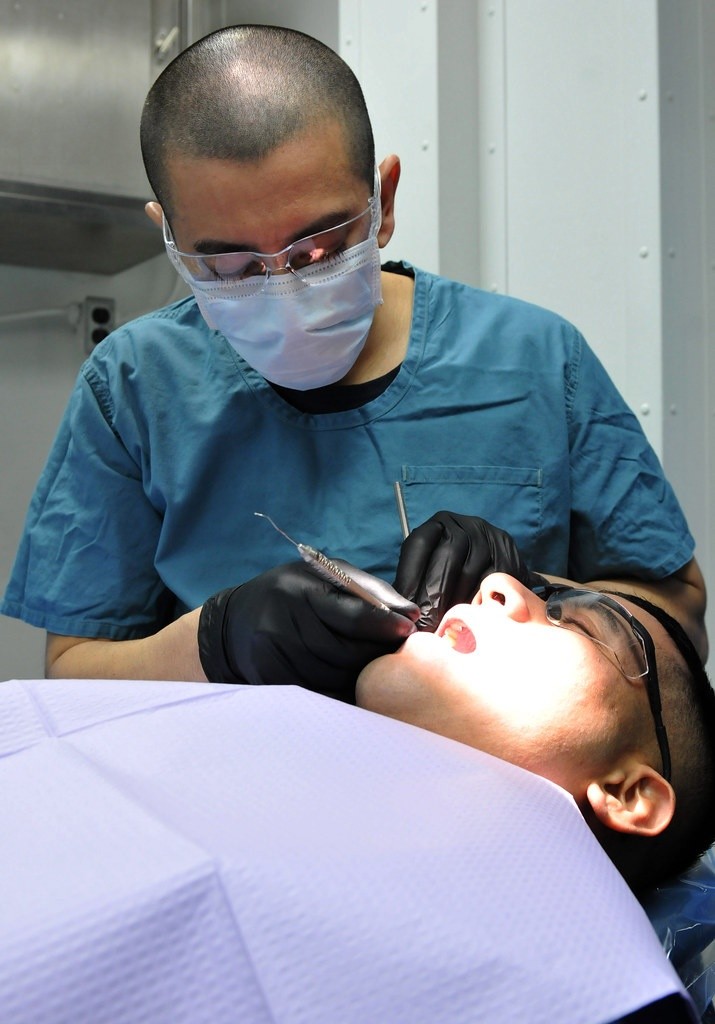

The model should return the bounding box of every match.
[0,572,715,1024]
[0,22,715,1024]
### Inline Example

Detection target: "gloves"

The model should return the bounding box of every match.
[393,510,553,633]
[198,558,408,706]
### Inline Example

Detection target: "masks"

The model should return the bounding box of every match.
[161,158,380,392]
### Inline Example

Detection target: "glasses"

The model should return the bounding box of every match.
[161,158,381,299]
[529,581,672,785]
